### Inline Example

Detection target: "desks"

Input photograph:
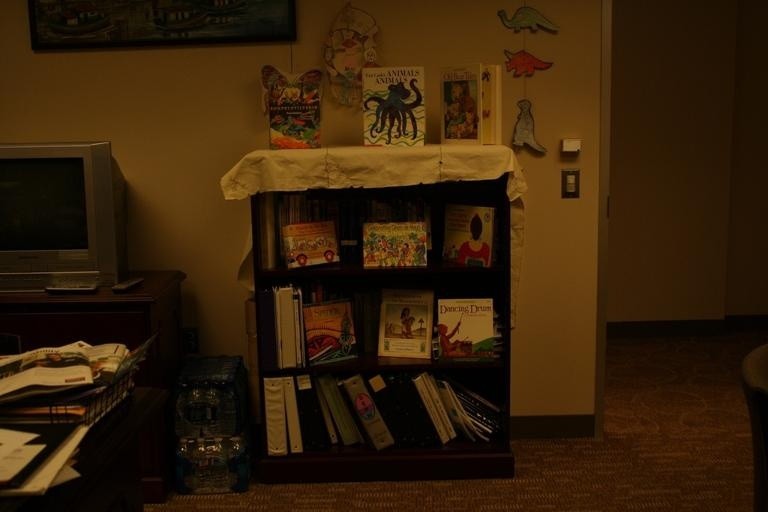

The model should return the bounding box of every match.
[0,387,182,510]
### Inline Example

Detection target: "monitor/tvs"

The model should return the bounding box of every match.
[0,142,127,293]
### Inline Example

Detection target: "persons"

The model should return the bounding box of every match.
[24,353,86,369]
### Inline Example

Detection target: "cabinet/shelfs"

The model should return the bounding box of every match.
[0,267,192,424]
[238,146,520,477]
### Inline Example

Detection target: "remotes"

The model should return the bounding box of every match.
[112,278,145,293]
[45,284,96,295]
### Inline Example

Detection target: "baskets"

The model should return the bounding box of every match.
[49,373,128,427]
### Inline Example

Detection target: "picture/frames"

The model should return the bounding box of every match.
[27,1,301,56]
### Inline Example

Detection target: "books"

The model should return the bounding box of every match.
[260,63,506,144]
[263,371,500,456]
[270,282,499,369]
[277,191,496,269]
